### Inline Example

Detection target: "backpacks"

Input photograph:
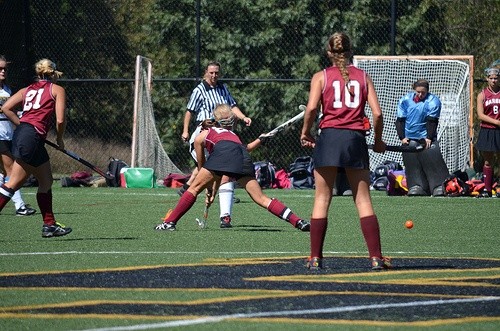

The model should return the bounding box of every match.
[71,169,93,187]
[105,156,129,187]
[290,155,316,190]
[253,160,276,189]
[163,172,192,189]
[372,160,403,190]
[271,169,291,189]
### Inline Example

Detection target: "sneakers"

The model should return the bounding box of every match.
[296,220,311,231]
[15,203,36,217]
[309,256,326,274]
[41,221,73,238]
[153,221,176,231]
[370,256,388,269]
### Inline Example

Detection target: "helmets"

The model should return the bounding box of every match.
[445,177,469,196]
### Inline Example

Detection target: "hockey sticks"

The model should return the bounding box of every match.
[266,105,307,135]
[195,190,212,228]
[305,138,427,153]
[44,140,116,181]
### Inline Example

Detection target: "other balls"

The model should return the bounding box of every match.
[406,221,413,228]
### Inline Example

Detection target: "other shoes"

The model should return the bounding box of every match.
[433,185,445,196]
[408,185,429,196]
[177,186,186,196]
[232,196,240,203]
[220,212,232,228]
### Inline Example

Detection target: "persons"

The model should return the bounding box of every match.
[394,79,449,197]
[153,104,311,231]
[476,68,500,199]
[177,60,252,204]
[300,33,392,271]
[0,55,37,217]
[0,59,72,238]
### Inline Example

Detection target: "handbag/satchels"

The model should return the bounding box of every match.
[119,167,158,189]
[386,169,408,196]
[62,177,73,187]
